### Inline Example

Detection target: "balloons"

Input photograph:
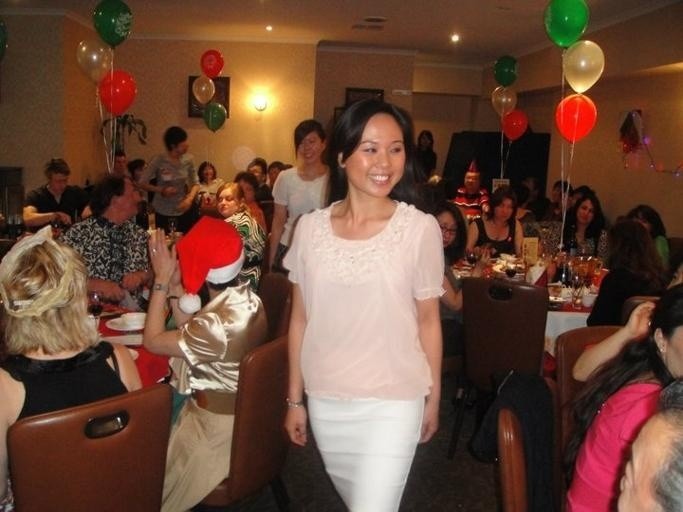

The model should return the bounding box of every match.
[192,48,228,134]
[542,0,606,145]
[77,0,137,117]
[490,54,528,141]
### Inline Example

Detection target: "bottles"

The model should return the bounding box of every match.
[51,218,59,239]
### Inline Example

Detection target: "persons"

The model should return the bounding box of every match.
[397,134,610,309]
[1,235,141,504]
[22,128,293,306]
[468,204,683,511]
[267,118,333,276]
[281,97,447,512]
[142,216,267,512]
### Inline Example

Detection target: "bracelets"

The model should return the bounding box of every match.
[284,397,305,409]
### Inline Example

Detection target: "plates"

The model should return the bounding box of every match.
[104,318,144,331]
[127,348,139,359]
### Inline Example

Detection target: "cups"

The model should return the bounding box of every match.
[462,245,604,311]
[121,312,146,326]
[87,291,104,317]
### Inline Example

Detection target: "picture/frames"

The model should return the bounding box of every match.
[188,76,229,119]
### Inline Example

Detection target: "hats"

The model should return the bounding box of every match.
[174,214,246,315]
